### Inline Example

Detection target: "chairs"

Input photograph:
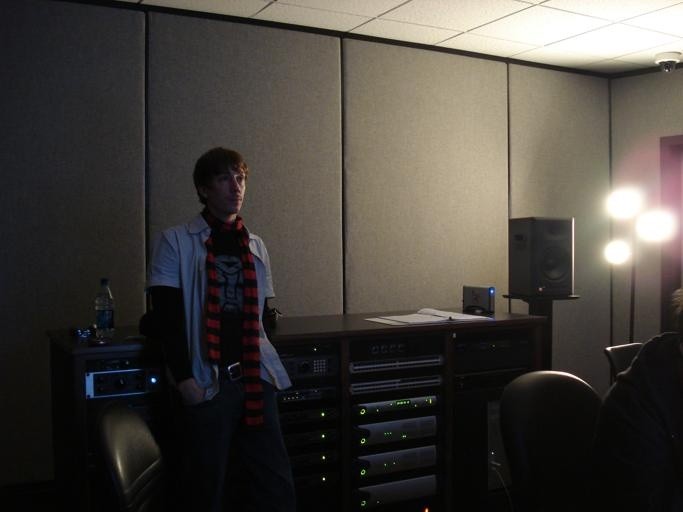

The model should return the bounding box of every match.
[89,398,173,511]
[496,366,604,511]
[601,339,644,384]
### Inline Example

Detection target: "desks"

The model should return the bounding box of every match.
[500,290,583,323]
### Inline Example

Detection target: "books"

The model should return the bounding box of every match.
[364,307,492,327]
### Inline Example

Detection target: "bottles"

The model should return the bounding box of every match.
[94,278,115,344]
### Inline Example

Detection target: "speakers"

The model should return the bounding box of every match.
[509,217,573,295]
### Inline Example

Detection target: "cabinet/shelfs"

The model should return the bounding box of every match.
[41,303,552,512]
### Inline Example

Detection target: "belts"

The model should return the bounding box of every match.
[218,358,246,384]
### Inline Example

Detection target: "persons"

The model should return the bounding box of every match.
[145,148,296,511]
[598,289,682,512]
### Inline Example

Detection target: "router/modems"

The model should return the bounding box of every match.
[462,284,495,314]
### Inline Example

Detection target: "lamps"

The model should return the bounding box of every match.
[599,181,678,345]
